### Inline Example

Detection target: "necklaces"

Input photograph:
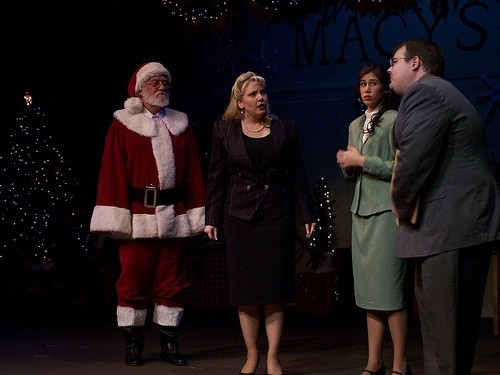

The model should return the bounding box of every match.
[242,120,265,132]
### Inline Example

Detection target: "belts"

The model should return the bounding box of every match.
[127,184,183,208]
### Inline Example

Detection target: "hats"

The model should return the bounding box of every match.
[124,62,171,113]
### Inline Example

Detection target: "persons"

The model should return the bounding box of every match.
[335,63,408,375]
[387,39,500,375]
[90,62,205,367]
[204,71,317,375]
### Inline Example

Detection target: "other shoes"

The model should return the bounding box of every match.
[362,363,386,375]
[390,363,412,375]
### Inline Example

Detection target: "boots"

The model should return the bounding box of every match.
[122,326,143,366]
[157,324,188,366]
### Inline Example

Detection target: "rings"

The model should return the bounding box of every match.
[340,160,343,164]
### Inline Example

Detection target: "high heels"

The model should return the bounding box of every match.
[266,351,283,375]
[239,354,261,375]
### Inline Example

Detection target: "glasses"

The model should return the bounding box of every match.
[149,79,169,86]
[390,58,423,66]
